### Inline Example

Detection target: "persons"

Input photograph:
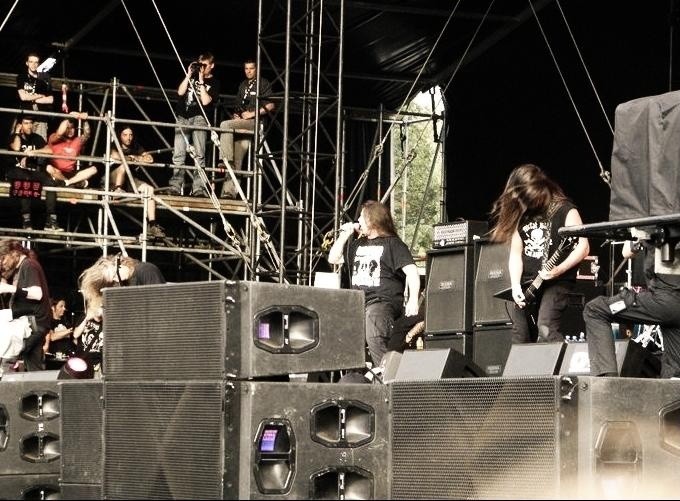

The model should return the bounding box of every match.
[219,57,276,200]
[580,226,680,379]
[0,239,166,383]
[325,198,423,387]
[167,53,221,200]
[16,53,56,132]
[99,126,168,239]
[8,114,64,239]
[46,112,98,189]
[484,162,590,345]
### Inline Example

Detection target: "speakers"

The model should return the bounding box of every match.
[2,478,59,501]
[422,245,473,335]
[471,242,525,324]
[56,483,101,501]
[503,339,564,376]
[102,280,368,383]
[106,380,388,501]
[559,337,662,376]
[61,379,105,483]
[389,377,680,500]
[469,325,515,375]
[426,334,465,377]
[0,371,60,475]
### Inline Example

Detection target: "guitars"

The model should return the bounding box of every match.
[492,236,579,313]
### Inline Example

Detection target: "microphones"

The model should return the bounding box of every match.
[339,224,362,236]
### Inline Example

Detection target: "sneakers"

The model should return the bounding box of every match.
[146,223,166,239]
[190,189,209,197]
[54,178,67,186]
[71,180,88,188]
[112,187,127,201]
[223,192,234,198]
[217,161,236,169]
[167,185,180,194]
